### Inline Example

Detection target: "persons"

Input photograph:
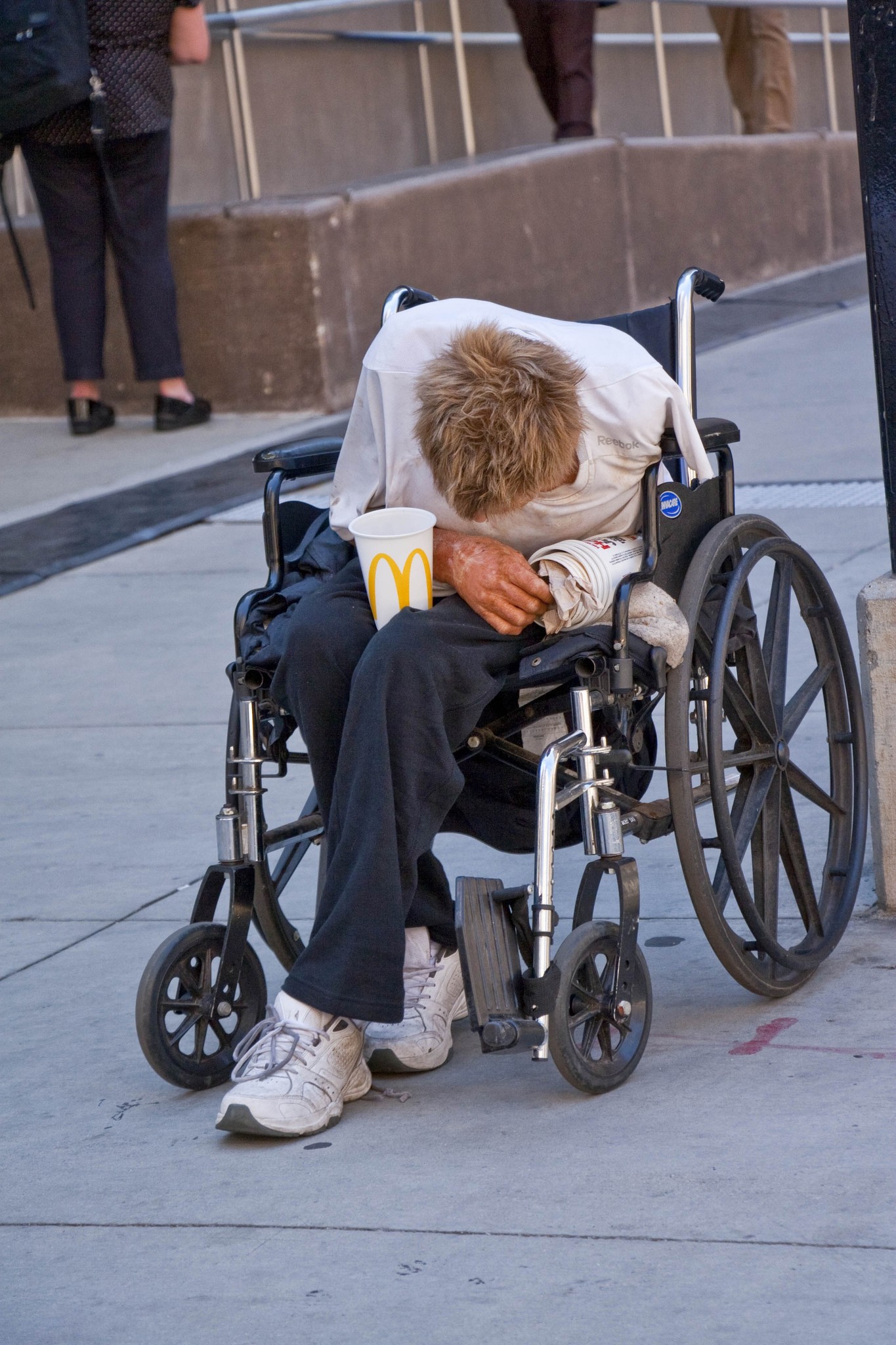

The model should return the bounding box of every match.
[707,5,801,138]
[23,1,210,437]
[216,298,704,1140]
[506,0,600,142]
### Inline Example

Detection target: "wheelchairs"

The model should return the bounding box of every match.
[133,262,865,1097]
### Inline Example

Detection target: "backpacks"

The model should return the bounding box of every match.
[1,1,93,135]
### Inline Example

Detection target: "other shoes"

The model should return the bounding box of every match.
[66,398,114,433]
[153,396,210,430]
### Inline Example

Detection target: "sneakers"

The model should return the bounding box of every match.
[215,992,372,1140]
[362,927,468,1075]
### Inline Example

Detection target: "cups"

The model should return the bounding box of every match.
[528,534,643,631]
[347,506,437,630]
